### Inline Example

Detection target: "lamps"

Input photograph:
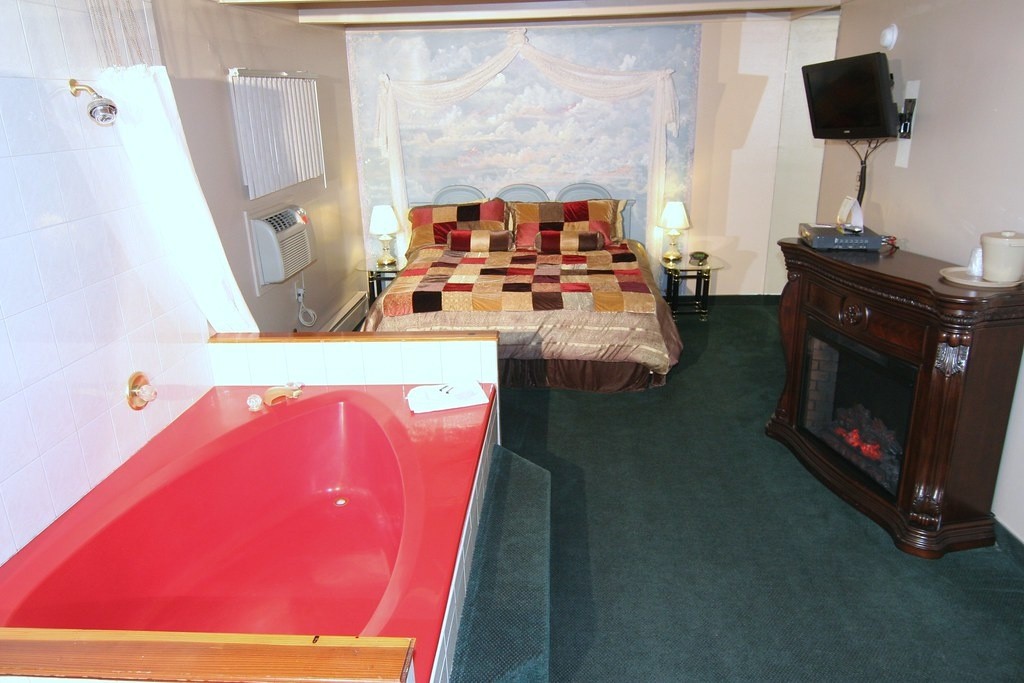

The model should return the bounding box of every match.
[369,205,400,265]
[658,202,690,261]
[126,372,158,410]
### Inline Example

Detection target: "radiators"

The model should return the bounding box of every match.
[227,67,327,200]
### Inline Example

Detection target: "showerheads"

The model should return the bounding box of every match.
[68,77,118,128]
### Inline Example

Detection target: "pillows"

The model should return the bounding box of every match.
[534,230,605,254]
[447,229,514,252]
[404,198,510,260]
[409,220,506,253]
[516,220,613,249]
[507,199,628,250]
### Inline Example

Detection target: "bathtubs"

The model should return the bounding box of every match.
[0,384,500,683]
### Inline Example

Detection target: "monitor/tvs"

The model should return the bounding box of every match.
[803,53,899,139]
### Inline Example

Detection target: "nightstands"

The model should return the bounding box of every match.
[659,252,724,323]
[356,256,409,311]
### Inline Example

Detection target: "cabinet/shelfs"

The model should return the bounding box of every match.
[765,237,1024,560]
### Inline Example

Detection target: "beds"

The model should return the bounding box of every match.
[360,184,684,395]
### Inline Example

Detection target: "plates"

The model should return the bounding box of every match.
[939,267,1024,287]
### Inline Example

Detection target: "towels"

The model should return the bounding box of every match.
[404,377,490,414]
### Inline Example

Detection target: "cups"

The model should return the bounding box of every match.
[966,249,983,276]
[981,231,1024,283]
